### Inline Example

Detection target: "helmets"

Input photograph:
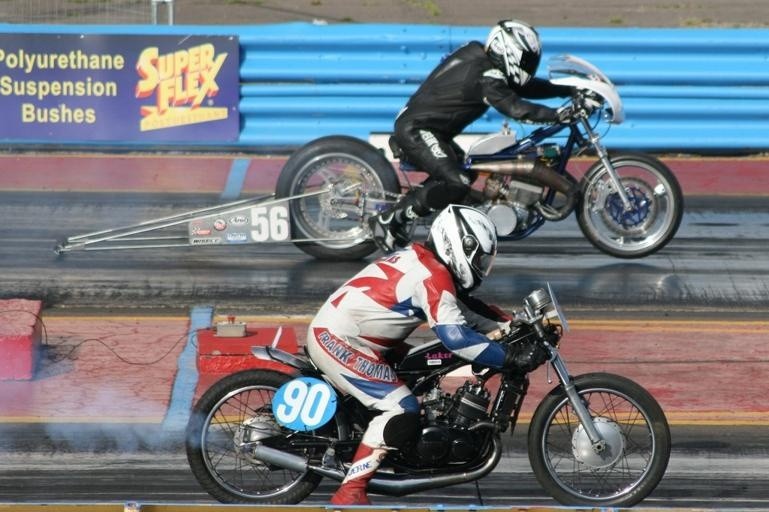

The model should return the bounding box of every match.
[424,204,499,294]
[485,18,542,90]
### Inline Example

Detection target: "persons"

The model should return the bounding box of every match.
[365,18,606,255]
[303,204,561,508]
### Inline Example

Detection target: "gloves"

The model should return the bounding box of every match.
[499,342,554,373]
[556,100,595,125]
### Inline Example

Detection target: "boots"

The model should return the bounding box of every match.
[367,192,427,255]
[328,442,388,506]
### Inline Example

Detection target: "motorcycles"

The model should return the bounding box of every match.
[182,276,675,509]
[264,52,687,266]
